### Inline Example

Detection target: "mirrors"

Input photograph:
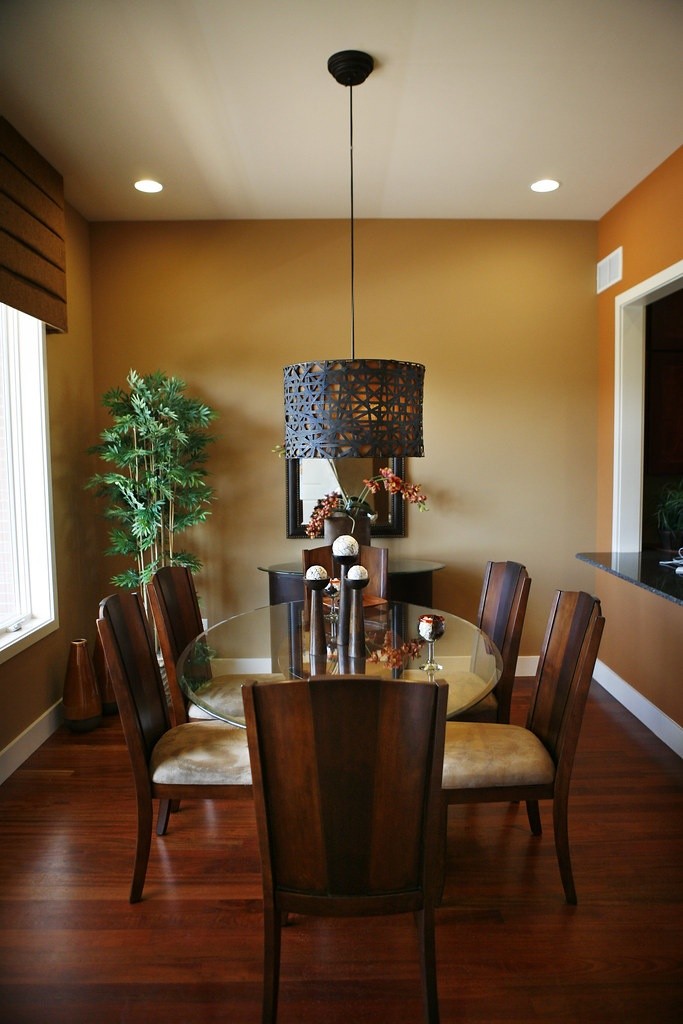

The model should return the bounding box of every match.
[287,455,405,539]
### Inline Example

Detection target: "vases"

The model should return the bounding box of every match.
[324,516,371,546]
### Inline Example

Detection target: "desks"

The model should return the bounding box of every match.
[177,601,504,725]
[258,559,447,609]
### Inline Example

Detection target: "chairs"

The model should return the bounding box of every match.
[241,673,450,1024]
[144,567,289,836]
[442,587,606,905]
[447,560,534,725]
[99,590,254,901]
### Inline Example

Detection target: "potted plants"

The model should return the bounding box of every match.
[646,481,683,558]
[84,365,221,704]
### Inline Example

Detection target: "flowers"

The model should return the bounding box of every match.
[303,467,428,539]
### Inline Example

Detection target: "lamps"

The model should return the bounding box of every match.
[283,50,426,458]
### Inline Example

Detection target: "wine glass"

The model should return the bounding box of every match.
[417,614,446,671]
